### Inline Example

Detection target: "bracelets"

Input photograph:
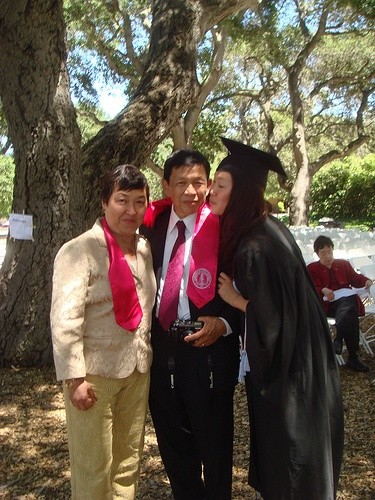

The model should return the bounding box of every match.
[65,378,85,387]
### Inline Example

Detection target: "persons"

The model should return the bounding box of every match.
[209,136,345,500]
[306,235,373,373]
[140,150,247,500]
[50,164,157,499]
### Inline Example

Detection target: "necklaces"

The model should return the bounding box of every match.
[121,236,134,257]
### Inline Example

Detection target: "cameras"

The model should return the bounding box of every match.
[169,319,203,345]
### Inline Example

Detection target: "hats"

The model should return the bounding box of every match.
[215,135,288,187]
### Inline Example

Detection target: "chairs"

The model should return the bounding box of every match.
[304,260,375,365]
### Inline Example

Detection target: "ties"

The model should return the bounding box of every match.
[156,221,186,331]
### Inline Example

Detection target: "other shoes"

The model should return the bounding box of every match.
[333,338,343,354]
[347,355,370,371]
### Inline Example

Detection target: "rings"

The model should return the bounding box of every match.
[201,344,205,348]
